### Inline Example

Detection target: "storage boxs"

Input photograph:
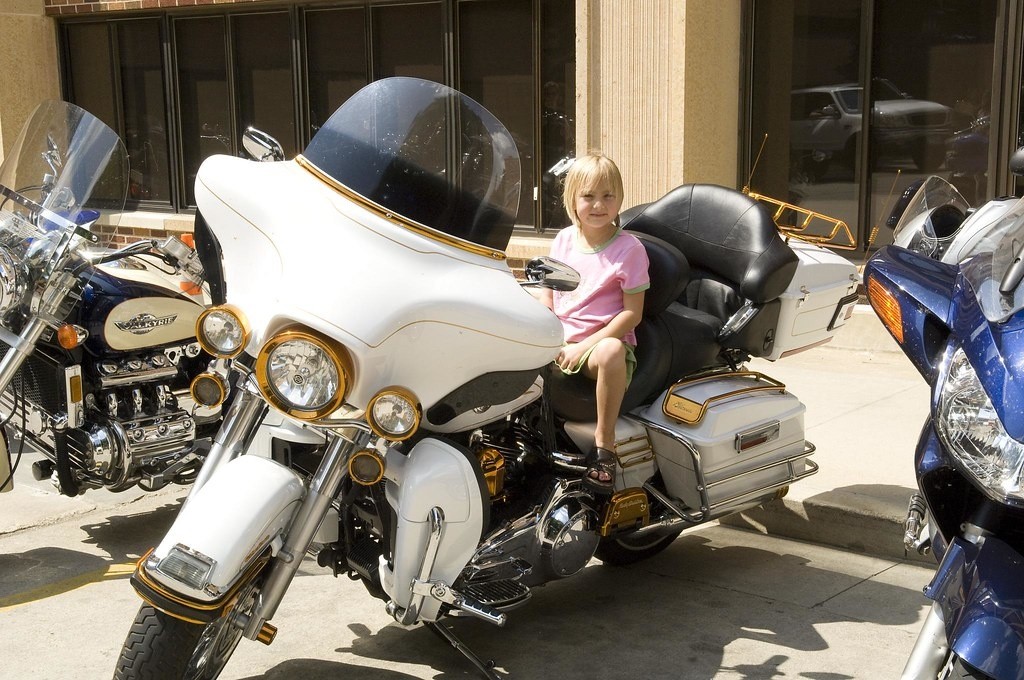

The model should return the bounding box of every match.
[764,232,861,361]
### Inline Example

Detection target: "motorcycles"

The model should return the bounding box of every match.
[863,144,1024,680]
[116,76,818,680]
[0,98,286,498]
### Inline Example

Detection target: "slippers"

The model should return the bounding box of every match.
[581,447,617,495]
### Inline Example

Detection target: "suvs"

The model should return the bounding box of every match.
[792,77,957,176]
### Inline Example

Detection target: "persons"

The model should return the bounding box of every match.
[533,154,651,491]
